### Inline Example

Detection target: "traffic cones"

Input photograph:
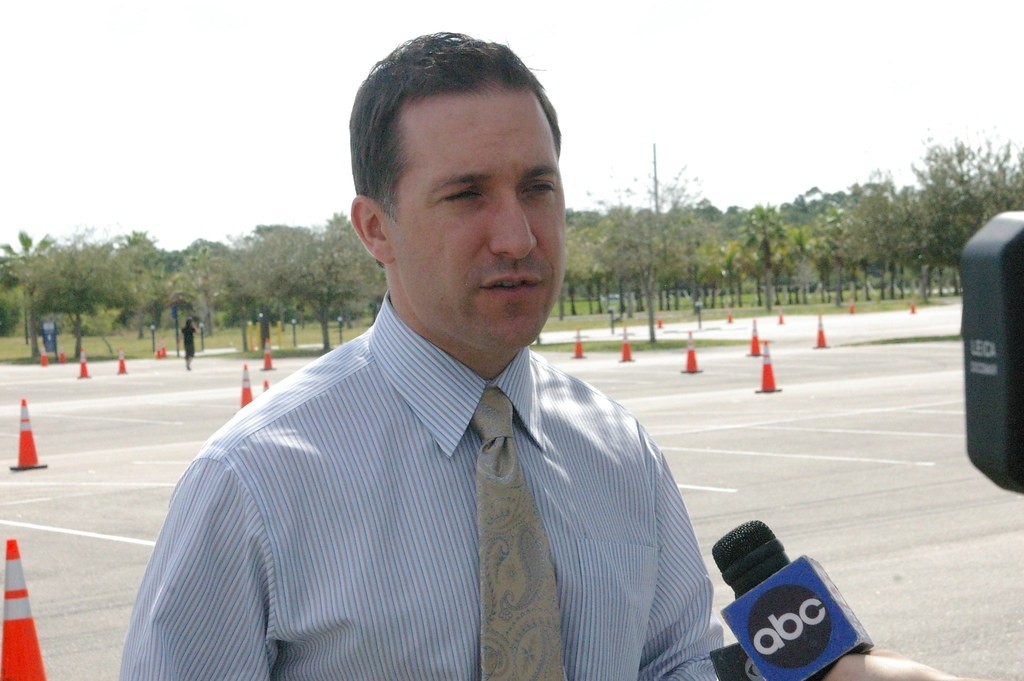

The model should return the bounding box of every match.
[241,364,252,408]
[78,348,93,380]
[264,380,269,392]
[726,311,735,324]
[155,335,167,359]
[1,538,49,681]
[909,302,918,314]
[618,334,635,363]
[814,315,832,349]
[848,296,855,314]
[40,344,48,366]
[58,340,67,363]
[570,326,586,359]
[681,332,702,373]
[116,345,128,375]
[11,398,47,470]
[754,340,783,392]
[747,319,763,357]
[655,315,664,329]
[262,337,277,371]
[776,305,785,324]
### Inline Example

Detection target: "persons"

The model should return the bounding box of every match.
[119,32,723,681]
[181,318,196,370]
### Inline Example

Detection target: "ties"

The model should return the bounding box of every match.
[469,386,566,681]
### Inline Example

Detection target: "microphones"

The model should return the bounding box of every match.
[711,518,874,681]
